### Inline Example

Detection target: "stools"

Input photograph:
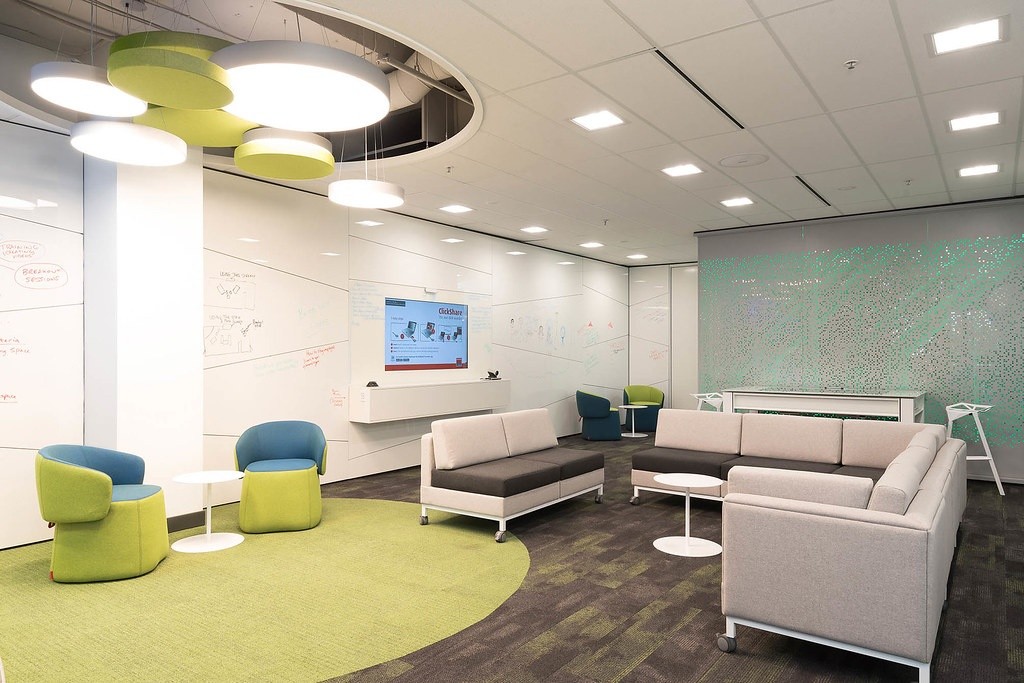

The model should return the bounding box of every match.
[688,392,723,412]
[945,402,1006,496]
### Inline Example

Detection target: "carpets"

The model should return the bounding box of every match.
[0,498,531,683]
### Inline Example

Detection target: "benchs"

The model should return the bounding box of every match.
[418,408,605,544]
[629,408,948,508]
[716,439,969,683]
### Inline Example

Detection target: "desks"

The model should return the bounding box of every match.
[652,472,724,558]
[171,470,246,554]
[618,405,648,438]
[720,385,927,423]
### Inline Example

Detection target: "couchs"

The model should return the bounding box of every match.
[35,444,170,585]
[622,385,665,433]
[233,420,328,534]
[575,389,623,441]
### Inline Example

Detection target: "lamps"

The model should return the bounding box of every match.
[29,0,406,209]
